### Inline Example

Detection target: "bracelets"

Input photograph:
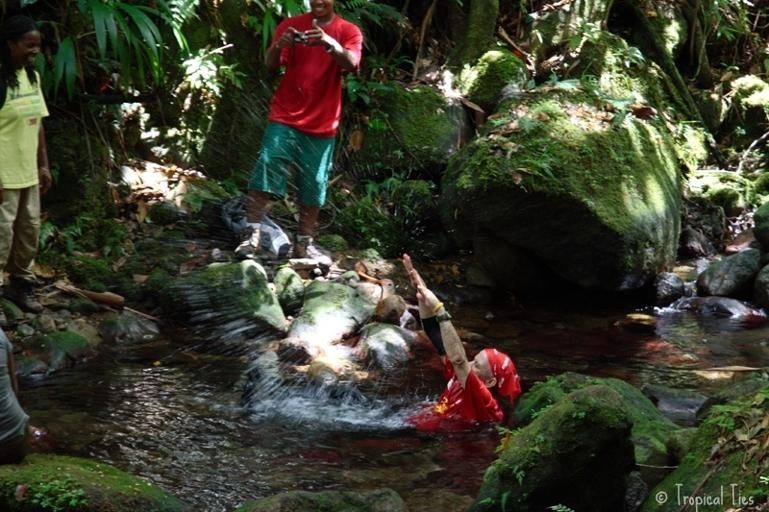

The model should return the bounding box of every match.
[432,302,444,314]
[436,312,452,322]
[326,45,334,52]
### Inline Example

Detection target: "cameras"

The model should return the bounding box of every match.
[294,32,309,43]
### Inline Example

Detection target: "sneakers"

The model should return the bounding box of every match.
[293,235,315,258]
[234,225,260,259]
[0,304,7,321]
[7,279,44,314]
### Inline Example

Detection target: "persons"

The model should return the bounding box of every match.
[234,0,363,268]
[403,253,522,434]
[0,327,48,464]
[0,15,52,313]
